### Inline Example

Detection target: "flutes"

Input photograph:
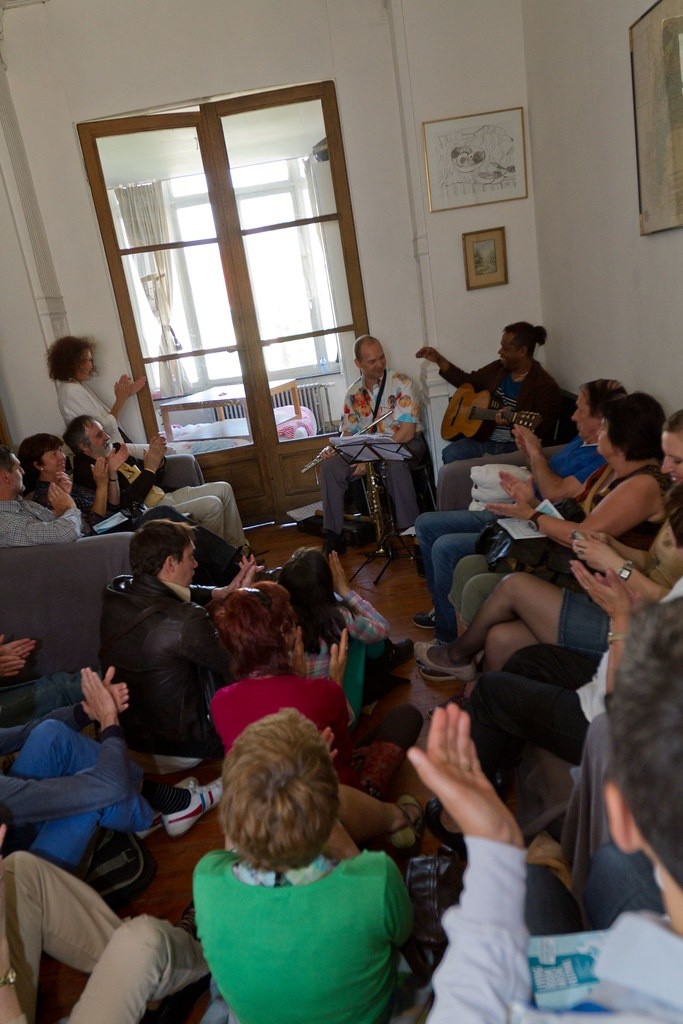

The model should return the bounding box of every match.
[300,410,396,476]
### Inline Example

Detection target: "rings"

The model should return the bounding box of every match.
[459,763,472,771]
[584,585,589,591]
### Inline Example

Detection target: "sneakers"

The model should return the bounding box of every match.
[172,899,197,939]
[161,777,222,836]
[427,694,468,719]
[135,778,198,838]
[412,607,436,629]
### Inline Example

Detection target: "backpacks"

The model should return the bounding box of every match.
[73,825,159,911]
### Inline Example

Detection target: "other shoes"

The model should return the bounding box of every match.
[235,554,265,566]
[425,797,467,861]
[322,529,347,560]
[390,793,425,857]
[370,638,414,672]
[414,639,475,680]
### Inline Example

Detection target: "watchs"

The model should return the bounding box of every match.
[527,511,546,532]
[616,560,633,581]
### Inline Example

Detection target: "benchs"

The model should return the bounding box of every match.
[0,451,202,680]
[438,441,587,838]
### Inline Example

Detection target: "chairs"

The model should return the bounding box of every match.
[354,433,436,529]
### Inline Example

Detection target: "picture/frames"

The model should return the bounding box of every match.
[462,225,509,290]
[423,106,527,214]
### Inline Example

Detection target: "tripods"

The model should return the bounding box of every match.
[333,442,416,585]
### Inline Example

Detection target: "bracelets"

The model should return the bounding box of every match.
[109,479,116,482]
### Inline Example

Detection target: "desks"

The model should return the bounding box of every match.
[161,377,301,443]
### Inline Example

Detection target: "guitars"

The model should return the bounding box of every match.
[440,382,544,444]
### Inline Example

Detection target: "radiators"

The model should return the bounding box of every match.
[206,382,334,434]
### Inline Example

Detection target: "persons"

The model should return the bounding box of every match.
[0,320,683,1024]
[44,336,179,459]
[316,335,425,576]
[407,596,683,1024]
[191,711,414,1024]
